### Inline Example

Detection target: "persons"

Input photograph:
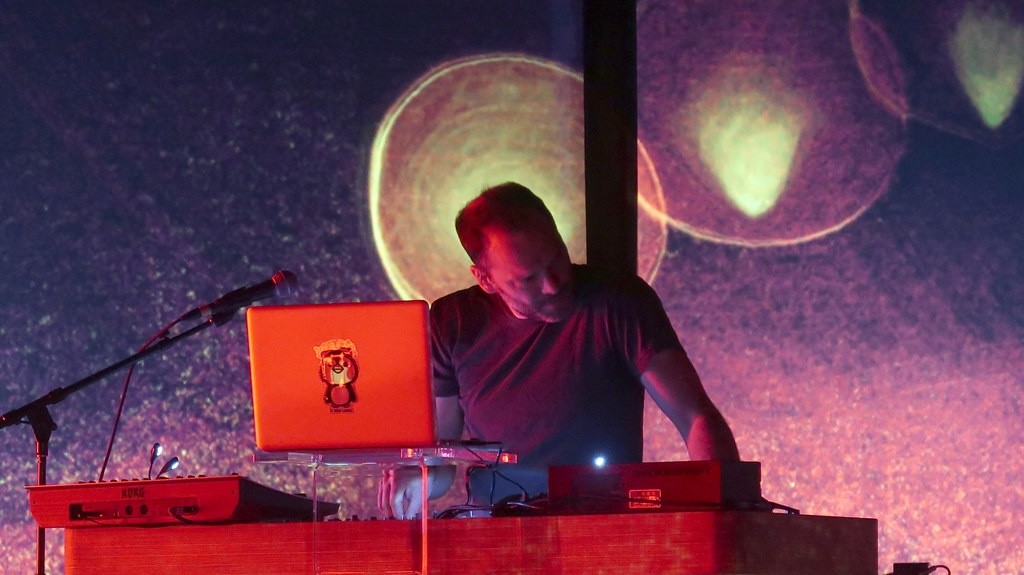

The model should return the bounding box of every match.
[375,181,764,521]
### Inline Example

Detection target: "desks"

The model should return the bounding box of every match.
[67,511,880,575]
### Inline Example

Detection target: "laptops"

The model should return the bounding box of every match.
[247,299,504,453]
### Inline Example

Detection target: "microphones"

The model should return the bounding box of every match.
[181,271,298,321]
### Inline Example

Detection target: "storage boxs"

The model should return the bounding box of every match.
[549,460,763,510]
